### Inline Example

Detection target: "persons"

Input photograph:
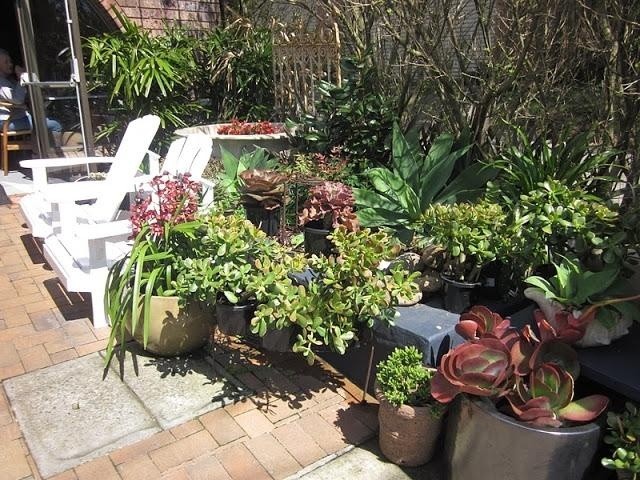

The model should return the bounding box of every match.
[0,47,63,135]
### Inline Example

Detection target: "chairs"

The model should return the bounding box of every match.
[42,134,215,330]
[0,101,62,176]
[19,114,162,240]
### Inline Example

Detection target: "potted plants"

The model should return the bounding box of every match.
[419,205,501,309]
[374,349,442,467]
[297,149,349,225]
[258,279,304,355]
[218,217,265,336]
[305,231,406,375]
[431,295,639,480]
[297,183,355,259]
[350,120,490,291]
[231,148,292,239]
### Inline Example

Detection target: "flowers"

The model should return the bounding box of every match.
[124,169,203,249]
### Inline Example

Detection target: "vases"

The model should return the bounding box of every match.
[122,288,216,357]
